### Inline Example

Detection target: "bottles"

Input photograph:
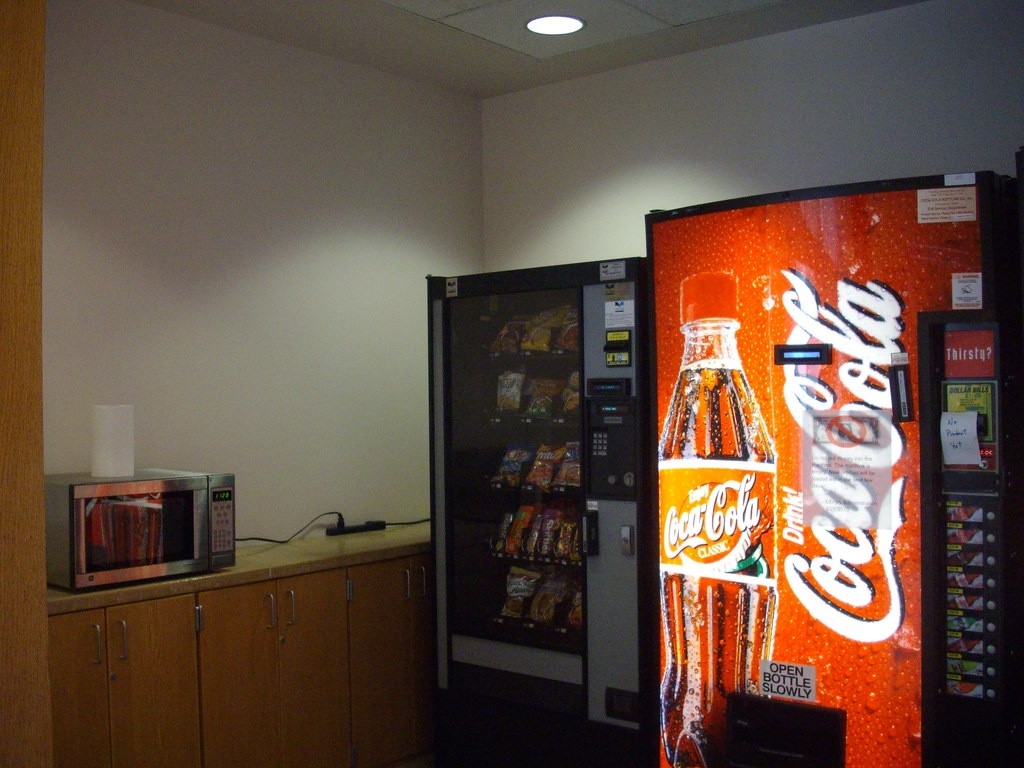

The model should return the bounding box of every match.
[656,271,778,768]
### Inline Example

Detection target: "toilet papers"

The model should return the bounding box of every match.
[90,403,135,477]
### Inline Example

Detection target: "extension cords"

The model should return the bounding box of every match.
[325,520,386,536]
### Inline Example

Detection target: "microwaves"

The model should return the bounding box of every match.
[45,468,240,593]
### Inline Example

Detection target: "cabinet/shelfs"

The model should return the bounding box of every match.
[348,555,439,767]
[197,566,352,768]
[48,593,201,767]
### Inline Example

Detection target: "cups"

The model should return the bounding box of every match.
[88,405,139,477]
